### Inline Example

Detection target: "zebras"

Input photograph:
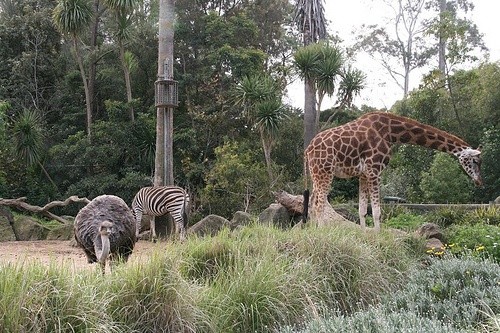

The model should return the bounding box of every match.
[131,185,189,243]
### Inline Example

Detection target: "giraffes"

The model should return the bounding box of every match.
[300,110,486,233]
